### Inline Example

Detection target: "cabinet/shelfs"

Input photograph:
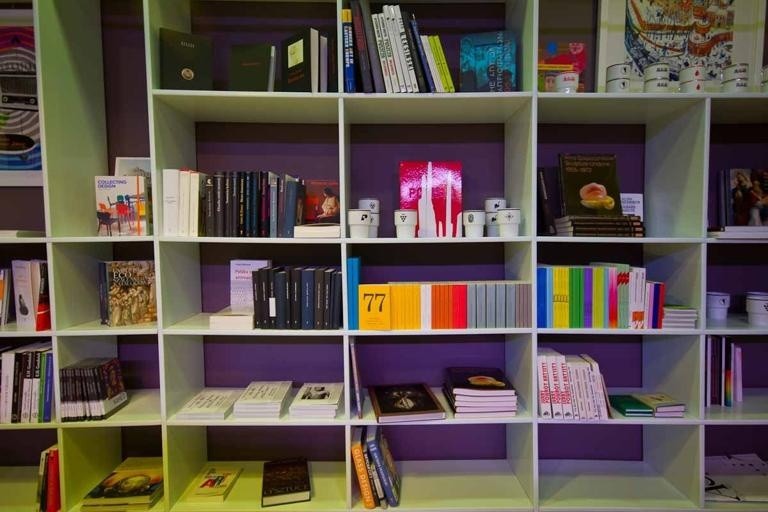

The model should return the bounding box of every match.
[1,0,768,512]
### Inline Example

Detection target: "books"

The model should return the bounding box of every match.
[35,443,61,512]
[1,340,55,425]
[706,169,768,239]
[176,380,345,421]
[705,335,742,407]
[442,366,518,418]
[537,262,698,330]
[94,175,150,236]
[536,346,613,419]
[610,392,686,419]
[188,467,243,503]
[1,259,51,331]
[59,357,127,423]
[159,27,328,93]
[350,339,364,418]
[460,30,517,92]
[162,169,340,238]
[369,382,446,423]
[81,471,164,512]
[348,256,532,330]
[352,425,403,509]
[209,259,342,330]
[342,1,455,93]
[261,458,311,507]
[537,153,644,238]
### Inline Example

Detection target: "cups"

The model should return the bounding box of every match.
[707,291,731,321]
[746,291,767,326]
[394,210,417,238]
[679,64,705,94]
[462,209,486,238]
[606,63,632,94]
[644,62,670,93]
[720,63,749,94]
[484,198,521,238]
[348,198,380,238]
[555,71,580,93]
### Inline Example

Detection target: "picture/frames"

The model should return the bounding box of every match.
[594,1,766,93]
